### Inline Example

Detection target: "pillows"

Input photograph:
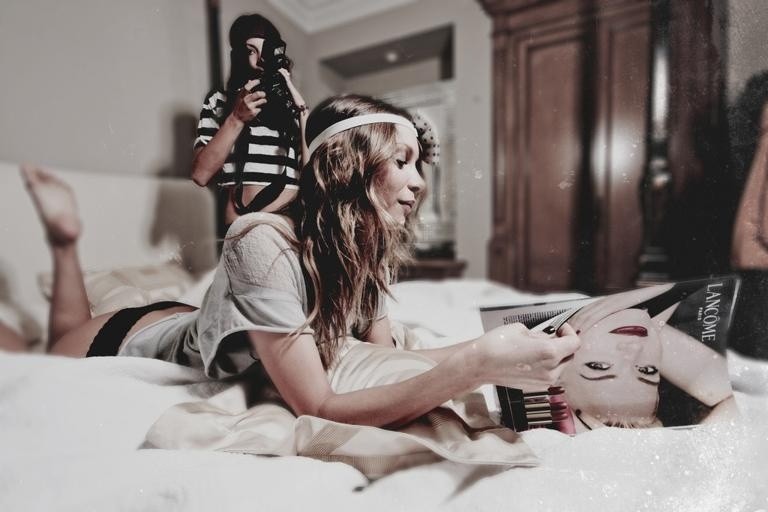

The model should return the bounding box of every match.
[145,332,540,472]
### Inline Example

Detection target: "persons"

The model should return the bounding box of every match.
[554,287,735,427]
[191,9,309,237]
[1,96,584,426]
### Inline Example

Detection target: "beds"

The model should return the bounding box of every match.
[0,165,768,512]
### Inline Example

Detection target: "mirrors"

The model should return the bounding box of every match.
[372,81,456,243]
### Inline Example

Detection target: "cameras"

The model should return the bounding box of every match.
[251,39,301,132]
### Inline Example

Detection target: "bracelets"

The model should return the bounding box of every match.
[298,103,308,113]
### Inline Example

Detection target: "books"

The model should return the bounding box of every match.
[479,275,742,430]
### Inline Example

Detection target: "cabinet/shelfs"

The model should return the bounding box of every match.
[478,3,730,295]
